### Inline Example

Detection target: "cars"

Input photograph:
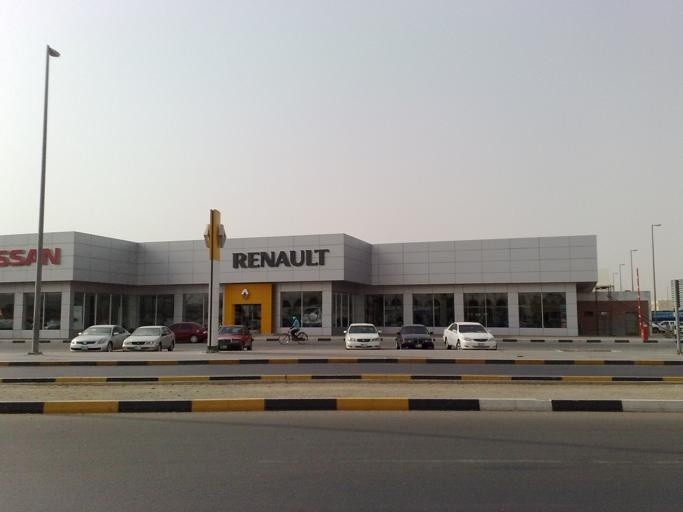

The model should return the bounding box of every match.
[396,325,434,349]
[69,324,130,351]
[170,322,208,343]
[443,322,497,350]
[122,325,176,351]
[217,325,253,351]
[678,322,683,333]
[344,323,381,350]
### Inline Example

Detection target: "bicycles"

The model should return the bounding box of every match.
[279,328,308,344]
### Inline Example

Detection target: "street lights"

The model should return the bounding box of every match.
[613,272,618,291]
[595,286,608,335]
[31,46,60,354]
[631,250,637,293]
[652,224,661,323]
[619,264,625,292]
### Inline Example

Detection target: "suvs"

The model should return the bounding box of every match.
[652,321,676,333]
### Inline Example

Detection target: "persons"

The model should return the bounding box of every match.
[291,316,300,340]
